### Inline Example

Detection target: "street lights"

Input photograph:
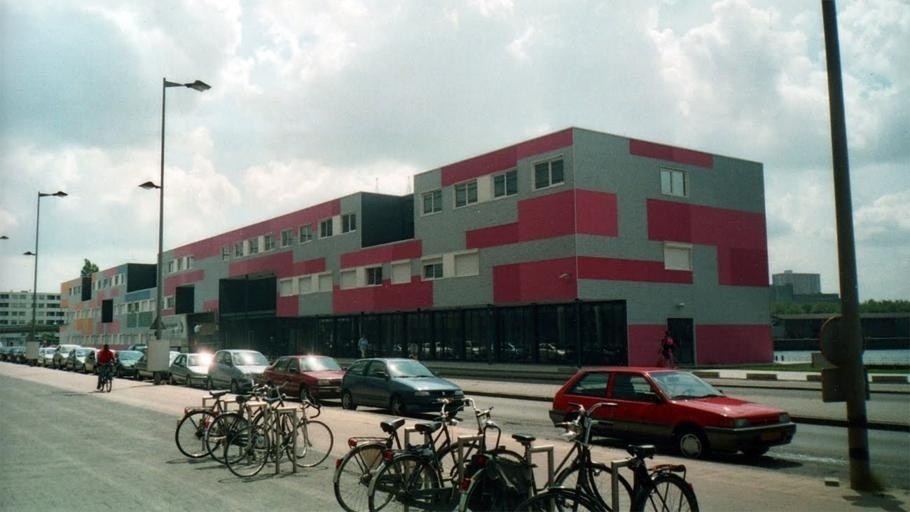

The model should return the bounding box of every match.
[22,189,70,339]
[135,74,214,387]
[0,235,10,240]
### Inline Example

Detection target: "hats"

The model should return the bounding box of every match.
[206,348,272,395]
[388,339,570,356]
[338,356,469,419]
[259,352,348,407]
[0,339,214,390]
[548,366,797,459]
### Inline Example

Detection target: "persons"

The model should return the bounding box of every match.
[358,336,368,357]
[661,331,673,367]
[97,345,115,389]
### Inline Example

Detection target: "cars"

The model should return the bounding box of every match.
[548,366,797,459]
[338,356,469,419]
[0,339,214,390]
[259,352,348,407]
[388,339,570,356]
[206,348,272,395]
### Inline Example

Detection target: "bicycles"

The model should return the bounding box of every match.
[94,360,116,393]
[172,376,337,478]
[332,398,479,512]
[367,398,527,512]
[514,419,700,512]
[454,401,636,512]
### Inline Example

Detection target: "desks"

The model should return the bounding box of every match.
[135,74,214,387]
[0,235,10,240]
[22,189,70,339]
